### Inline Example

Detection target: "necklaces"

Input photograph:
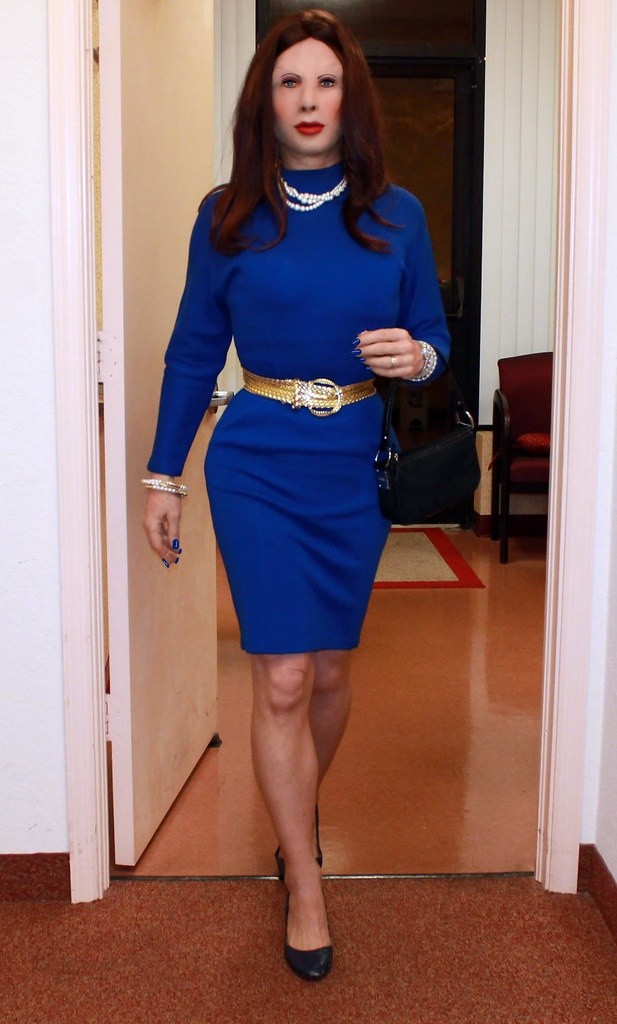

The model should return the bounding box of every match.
[278,176,348,212]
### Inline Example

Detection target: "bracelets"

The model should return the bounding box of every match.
[411,339,436,383]
[141,479,188,498]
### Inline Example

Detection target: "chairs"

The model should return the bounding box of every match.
[490,352,553,564]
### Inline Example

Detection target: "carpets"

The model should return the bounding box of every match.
[373,527,485,589]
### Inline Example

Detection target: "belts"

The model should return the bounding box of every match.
[241,368,377,417]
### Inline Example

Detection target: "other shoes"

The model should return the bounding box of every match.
[274,806,322,882]
[283,893,332,980]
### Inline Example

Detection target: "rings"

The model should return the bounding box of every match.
[391,355,397,369]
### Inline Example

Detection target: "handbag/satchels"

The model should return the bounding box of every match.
[374,348,480,526]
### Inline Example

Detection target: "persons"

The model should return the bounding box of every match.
[139,7,450,979]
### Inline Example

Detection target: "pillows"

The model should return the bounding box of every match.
[520,433,550,455]
